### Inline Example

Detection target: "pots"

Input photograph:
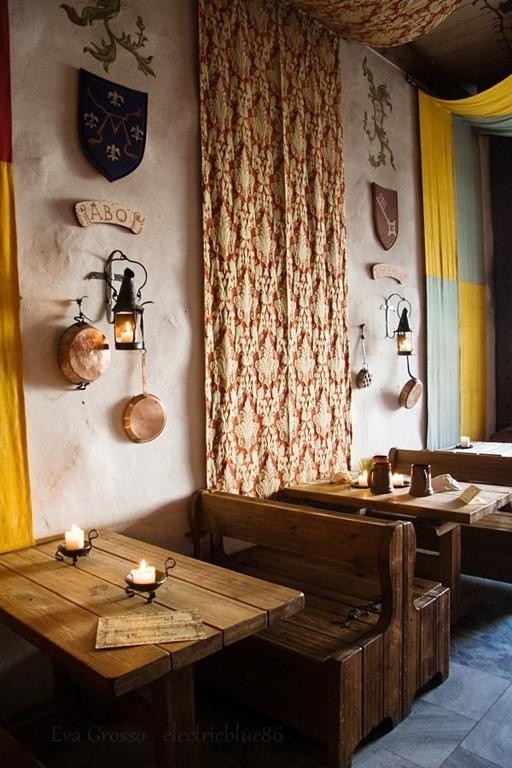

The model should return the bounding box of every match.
[55,315,111,391]
[124,348,168,443]
[399,352,423,410]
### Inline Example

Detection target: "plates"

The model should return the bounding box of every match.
[351,480,368,488]
[392,481,410,487]
[459,444,472,449]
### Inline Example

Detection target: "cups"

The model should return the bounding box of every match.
[367,460,395,496]
[372,453,388,465]
[459,437,470,447]
[408,462,436,498]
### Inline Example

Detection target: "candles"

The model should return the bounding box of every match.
[393,473,404,484]
[65,530,85,548]
[359,475,368,486]
[459,435,469,448]
[128,564,157,584]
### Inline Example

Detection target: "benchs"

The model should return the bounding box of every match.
[365,449,512,587]
[184,490,404,767]
[490,426,512,442]
[215,491,450,713]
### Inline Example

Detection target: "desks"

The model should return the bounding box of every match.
[453,439,511,459]
[281,472,511,615]
[0,531,306,763]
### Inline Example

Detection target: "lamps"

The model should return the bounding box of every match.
[106,248,153,348]
[386,293,415,357]
